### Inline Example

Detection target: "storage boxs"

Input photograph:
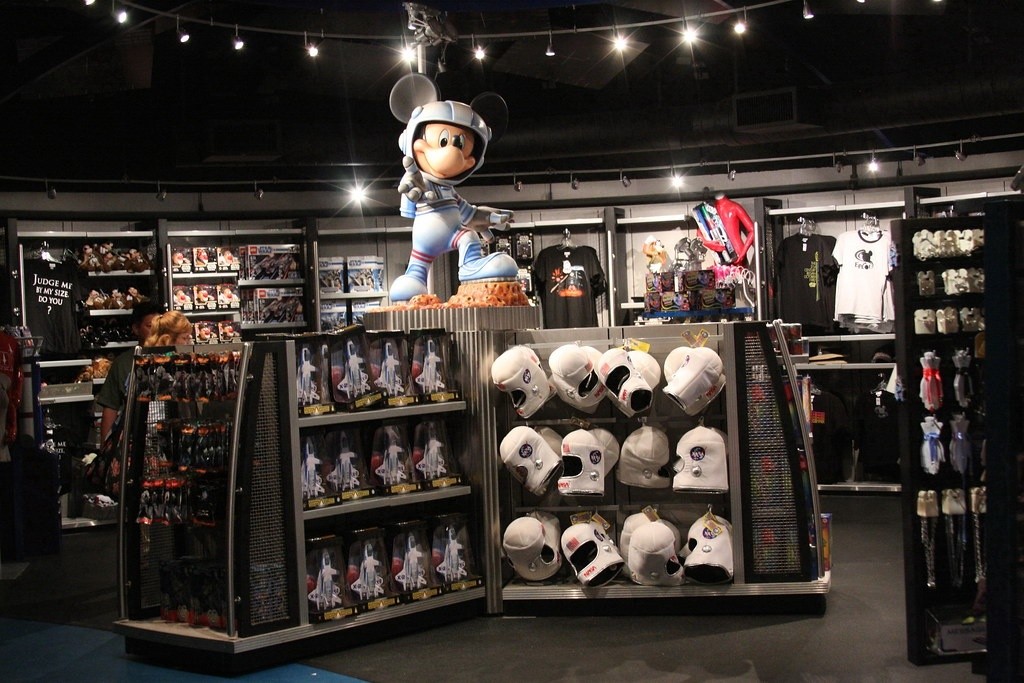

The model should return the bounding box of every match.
[239,246,246,280]
[245,245,302,281]
[810,513,835,570]
[330,323,388,411]
[408,327,461,402]
[246,288,255,324]
[241,289,248,325]
[362,422,424,496]
[254,287,305,323]
[367,328,419,406]
[380,516,444,604]
[284,334,337,419]
[298,428,342,510]
[173,244,243,342]
[317,427,376,501]
[306,532,359,623]
[341,526,403,615]
[426,512,485,594]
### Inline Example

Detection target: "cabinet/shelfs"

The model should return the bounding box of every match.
[792,360,903,492]
[756,185,942,344]
[496,318,835,618]
[5,216,168,389]
[136,216,322,333]
[105,329,486,676]
[889,216,989,666]
[606,196,785,319]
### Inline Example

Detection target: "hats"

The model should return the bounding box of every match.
[491,343,734,585]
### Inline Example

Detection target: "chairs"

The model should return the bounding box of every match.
[408,418,463,492]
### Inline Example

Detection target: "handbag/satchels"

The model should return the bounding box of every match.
[81,409,126,502]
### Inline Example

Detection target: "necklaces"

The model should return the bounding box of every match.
[918,508,987,591]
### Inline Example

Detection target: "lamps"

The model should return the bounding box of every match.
[176,14,190,43]
[726,161,737,182]
[304,32,319,57]
[253,181,265,200]
[155,181,168,202]
[471,33,485,59]
[955,140,968,162]
[569,171,581,191]
[230,24,244,50]
[619,169,632,188]
[45,180,56,200]
[513,173,522,193]
[832,153,843,173]
[870,149,878,172]
[111,0,126,23]
[912,146,926,167]
[544,30,556,56]
[803,0,814,19]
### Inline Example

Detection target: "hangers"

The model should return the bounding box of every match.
[33,242,50,260]
[807,380,822,397]
[795,217,816,237]
[869,373,889,393]
[859,212,880,232]
[558,230,578,251]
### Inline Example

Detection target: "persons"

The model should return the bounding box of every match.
[695,193,756,266]
[97,301,165,450]
[144,310,192,346]
